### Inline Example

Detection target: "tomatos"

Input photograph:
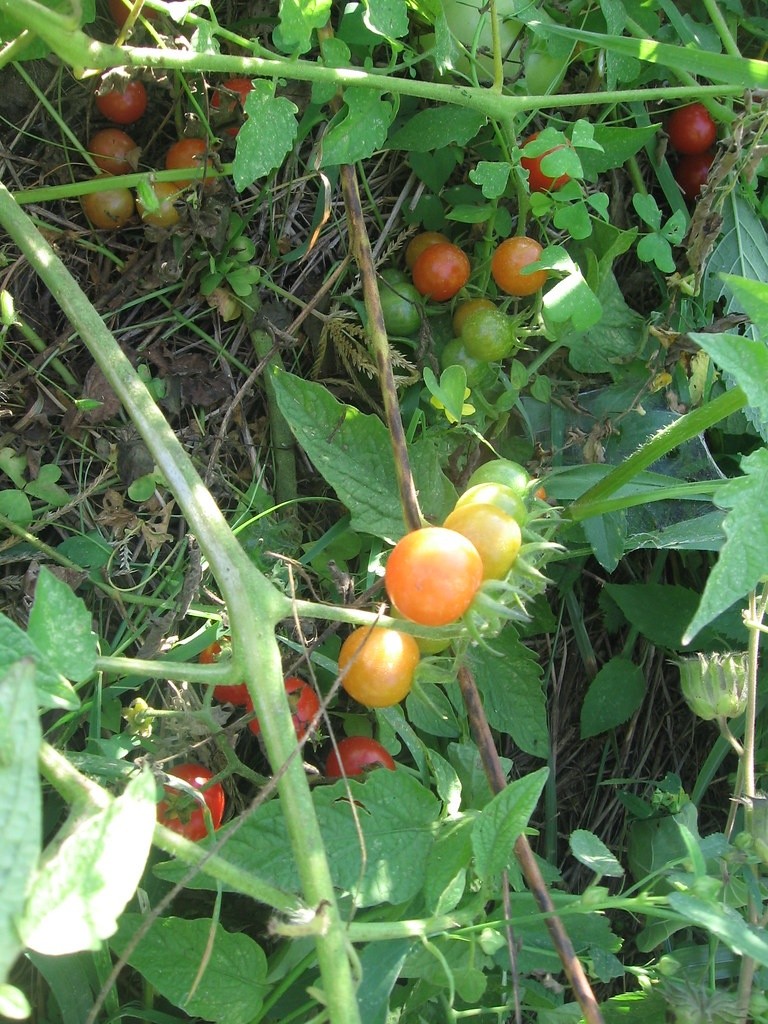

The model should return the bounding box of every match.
[81,0,721,840]
